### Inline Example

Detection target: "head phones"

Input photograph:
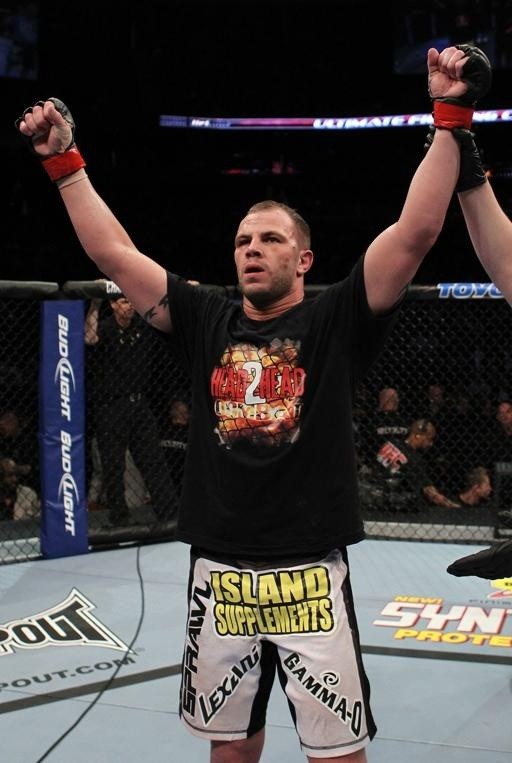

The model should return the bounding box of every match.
[417,419,429,433]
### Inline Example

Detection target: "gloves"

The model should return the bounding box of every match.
[449,540,512,581]
[424,122,486,195]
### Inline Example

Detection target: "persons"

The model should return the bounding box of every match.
[352,298,511,512]
[422,114,511,581]
[1,279,192,521]
[10,34,494,763]
